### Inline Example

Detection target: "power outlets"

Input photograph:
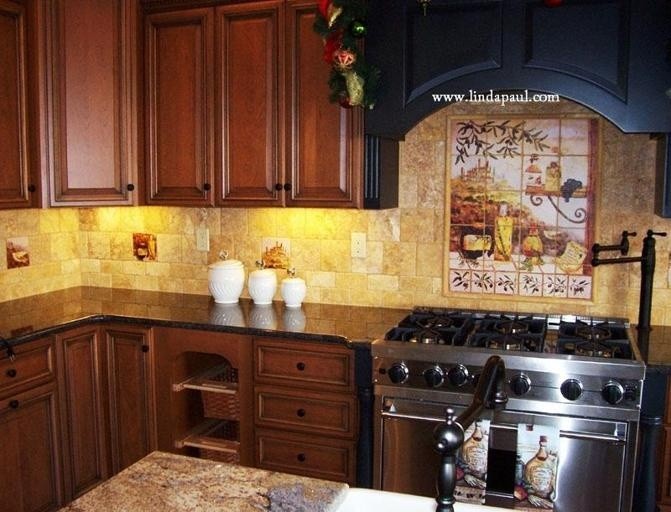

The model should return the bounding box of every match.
[351,231,367,258]
[196,229,210,253]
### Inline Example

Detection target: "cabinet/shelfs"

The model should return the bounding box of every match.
[101,323,154,479]
[1,0,36,210]
[212,0,363,209]
[42,1,145,206]
[153,326,356,484]
[1,319,109,512]
[140,0,216,205]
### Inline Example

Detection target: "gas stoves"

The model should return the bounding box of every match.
[372,306,646,422]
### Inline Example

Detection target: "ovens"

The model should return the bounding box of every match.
[372,394,643,512]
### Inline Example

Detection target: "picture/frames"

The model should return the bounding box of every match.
[442,113,599,309]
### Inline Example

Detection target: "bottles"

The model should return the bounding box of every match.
[137,242,148,257]
[461,422,489,478]
[148,236,156,260]
[495,154,562,261]
[524,435,555,498]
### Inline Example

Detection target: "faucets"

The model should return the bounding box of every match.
[433,355,508,511]
[619,230,637,257]
